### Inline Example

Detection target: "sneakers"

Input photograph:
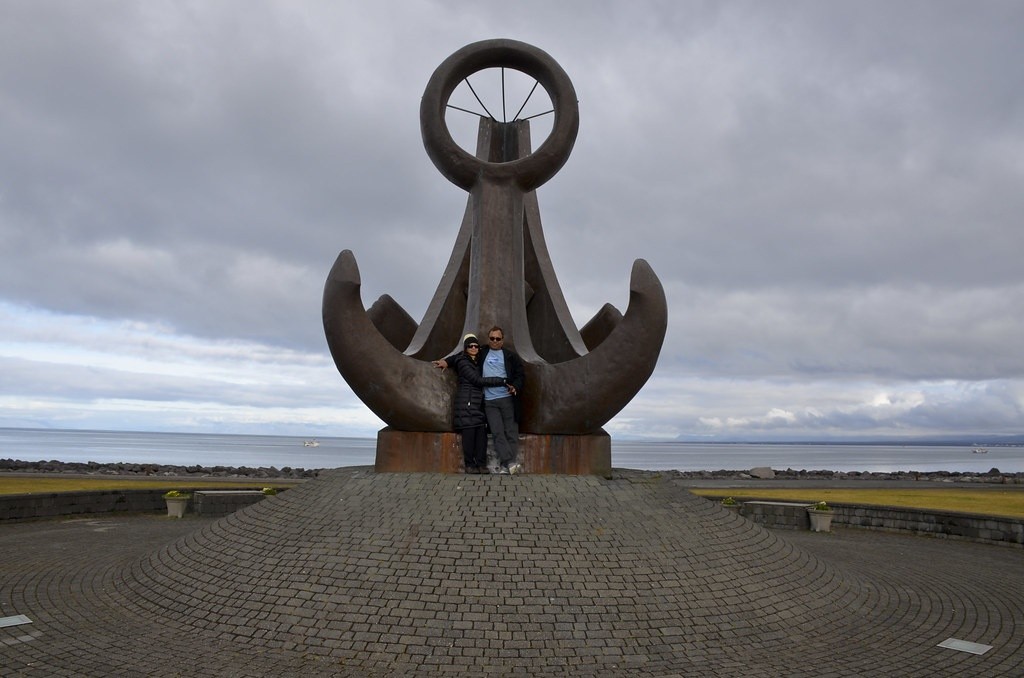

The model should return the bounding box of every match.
[500,462,521,475]
[465,466,490,474]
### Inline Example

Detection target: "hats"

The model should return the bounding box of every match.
[463,333,480,351]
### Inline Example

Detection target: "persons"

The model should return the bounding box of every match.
[432,326,526,475]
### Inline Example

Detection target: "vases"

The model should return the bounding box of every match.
[166,498,189,518]
[807,509,834,532]
[265,494,273,498]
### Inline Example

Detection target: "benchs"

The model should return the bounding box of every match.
[194,491,265,516]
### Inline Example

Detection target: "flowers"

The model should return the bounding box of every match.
[263,487,277,495]
[162,491,191,498]
[807,501,832,512]
[719,498,740,508]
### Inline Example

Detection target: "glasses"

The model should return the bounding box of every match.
[467,345,478,348]
[489,337,503,341]
